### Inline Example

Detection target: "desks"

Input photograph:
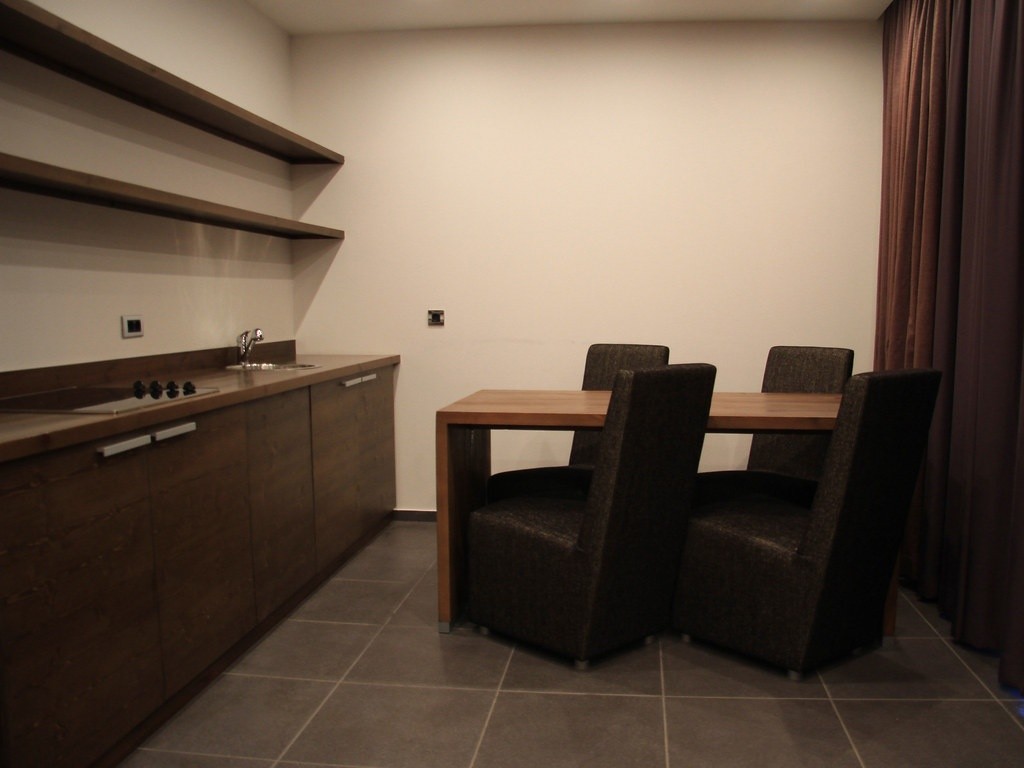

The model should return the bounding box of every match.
[436,390,898,638]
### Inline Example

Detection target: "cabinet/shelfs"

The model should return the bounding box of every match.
[0,0,344,240]
[0,358,395,768]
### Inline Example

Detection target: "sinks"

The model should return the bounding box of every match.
[224,362,322,371]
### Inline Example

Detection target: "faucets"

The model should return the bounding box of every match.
[240,328,264,365]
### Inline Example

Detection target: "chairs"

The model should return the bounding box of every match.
[469,344,943,682]
